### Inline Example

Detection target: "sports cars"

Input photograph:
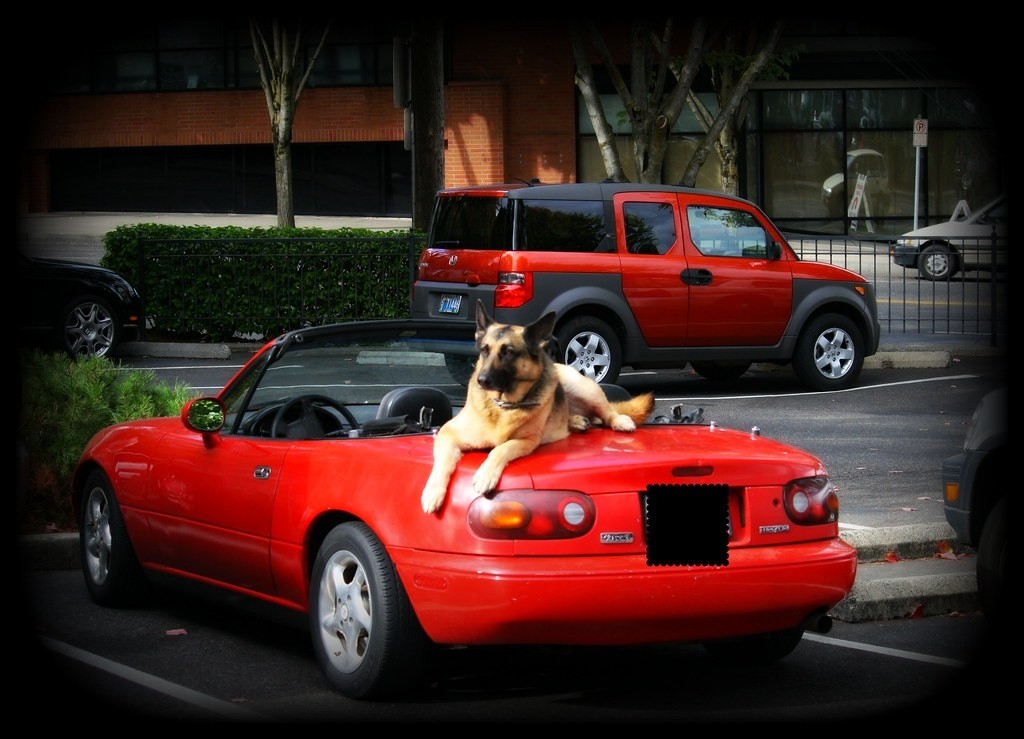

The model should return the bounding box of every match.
[68,321,860,704]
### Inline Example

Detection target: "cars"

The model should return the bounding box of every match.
[29,255,148,366]
[822,147,889,217]
[888,196,995,282]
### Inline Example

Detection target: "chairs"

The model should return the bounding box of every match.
[376,387,455,429]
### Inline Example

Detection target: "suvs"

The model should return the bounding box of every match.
[415,180,885,395]
[939,384,1014,623]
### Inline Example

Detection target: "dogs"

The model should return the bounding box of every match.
[420,298,656,515]
